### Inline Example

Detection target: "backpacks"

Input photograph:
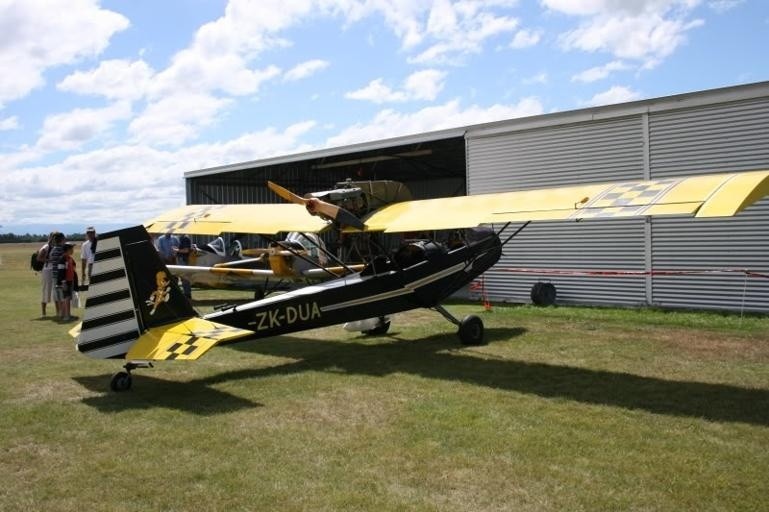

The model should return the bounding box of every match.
[30,250,44,272]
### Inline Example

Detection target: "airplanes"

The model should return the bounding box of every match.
[160,231,371,300]
[70,170,769,394]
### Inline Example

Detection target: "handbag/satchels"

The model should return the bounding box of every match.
[72,291,81,308]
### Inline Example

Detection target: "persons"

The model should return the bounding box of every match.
[81,226,97,286]
[158,231,178,288]
[170,233,193,301]
[50,232,74,320]
[55,243,81,322]
[35,231,64,320]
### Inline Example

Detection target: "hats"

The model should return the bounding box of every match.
[64,242,76,252]
[86,226,94,234]
